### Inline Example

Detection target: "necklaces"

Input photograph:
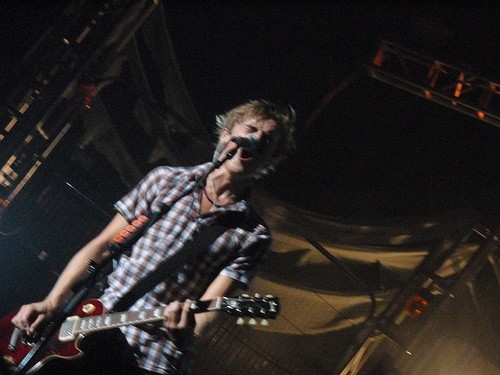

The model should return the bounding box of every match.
[203,188,221,208]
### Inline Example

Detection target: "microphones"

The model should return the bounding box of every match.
[231,136,258,151]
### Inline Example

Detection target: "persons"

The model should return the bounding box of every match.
[11,97,297,375]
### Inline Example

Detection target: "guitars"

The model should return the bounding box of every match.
[0,292,281,375]
